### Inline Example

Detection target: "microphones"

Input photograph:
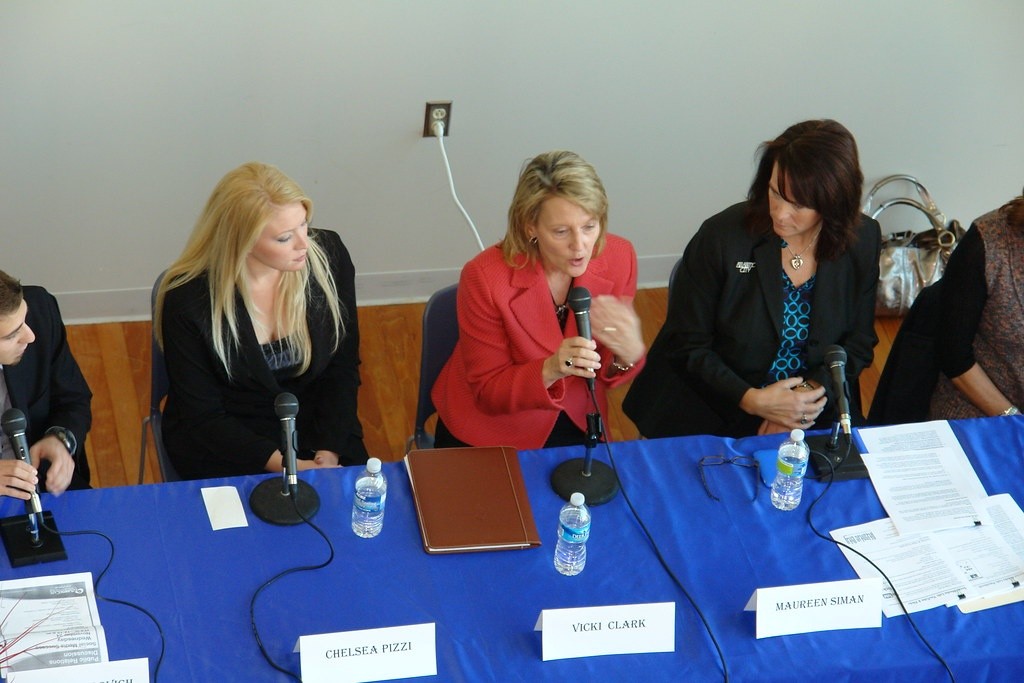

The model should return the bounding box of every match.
[567,286,594,392]
[275,392,298,500]
[822,343,852,445]
[1,408,45,524]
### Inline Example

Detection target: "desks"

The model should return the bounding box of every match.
[0,413,1024,683]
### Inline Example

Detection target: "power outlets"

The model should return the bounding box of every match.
[425,100,451,138]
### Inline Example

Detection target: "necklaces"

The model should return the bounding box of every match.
[786,225,822,272]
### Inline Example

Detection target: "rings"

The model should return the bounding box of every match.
[801,413,808,424]
[603,326,617,332]
[564,359,573,367]
[797,381,814,390]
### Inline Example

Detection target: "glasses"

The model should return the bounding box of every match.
[699,456,760,501]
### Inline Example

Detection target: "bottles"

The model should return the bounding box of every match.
[351,457,388,539]
[770,428,810,511]
[553,492,592,576]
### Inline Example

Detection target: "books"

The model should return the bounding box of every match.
[402,445,542,555]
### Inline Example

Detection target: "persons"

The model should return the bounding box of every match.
[929,186,1024,422]
[152,161,368,480]
[622,117,883,439]
[429,149,648,451]
[0,269,94,502]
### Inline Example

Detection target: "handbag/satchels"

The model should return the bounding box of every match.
[882,220,965,249]
[863,174,960,316]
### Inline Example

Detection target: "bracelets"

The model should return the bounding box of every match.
[998,404,1019,416]
[49,428,74,452]
[611,354,631,372]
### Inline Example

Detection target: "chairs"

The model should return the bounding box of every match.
[867,281,945,421]
[405,287,461,452]
[139,272,187,483]
